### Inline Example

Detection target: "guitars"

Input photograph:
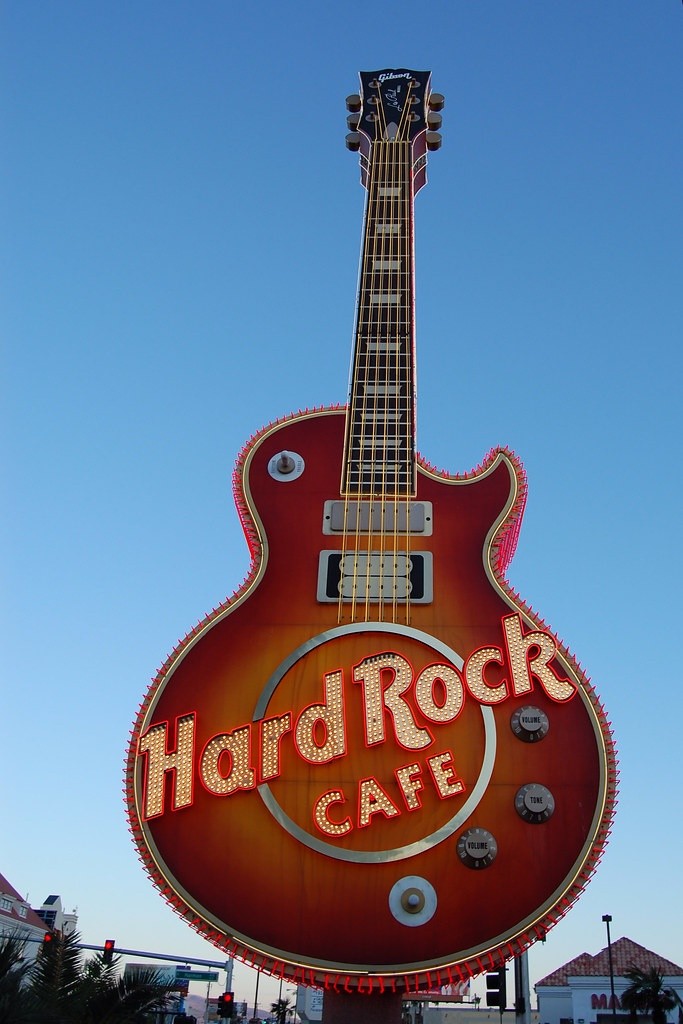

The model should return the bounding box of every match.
[122,67,620,994]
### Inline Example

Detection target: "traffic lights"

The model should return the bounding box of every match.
[217,992,233,1018]
[102,939,115,965]
[43,930,54,958]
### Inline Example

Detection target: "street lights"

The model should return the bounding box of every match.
[602,914,616,1022]
